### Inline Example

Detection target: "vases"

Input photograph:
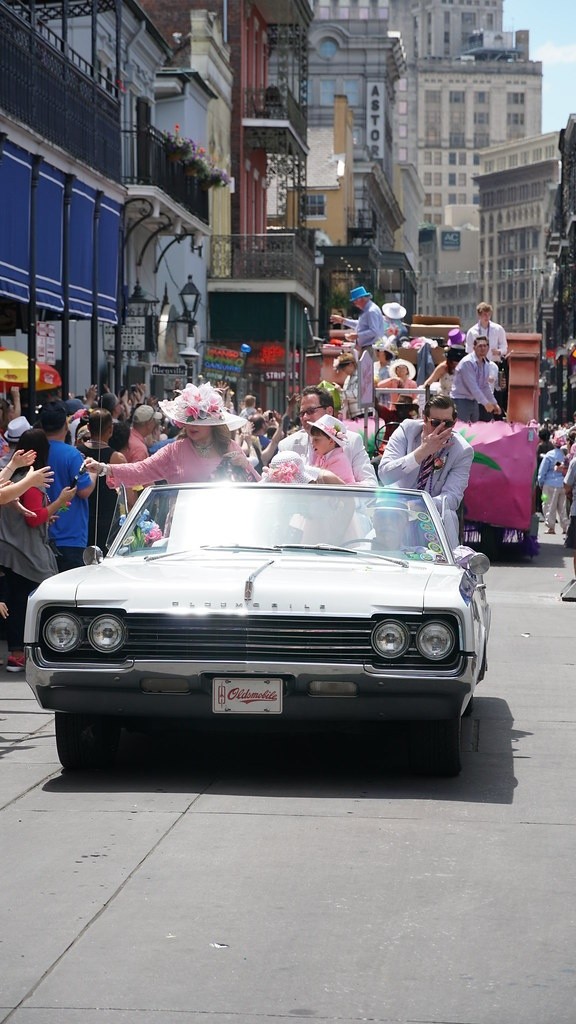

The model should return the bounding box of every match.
[185,167,196,176]
[168,152,180,163]
[202,180,214,192]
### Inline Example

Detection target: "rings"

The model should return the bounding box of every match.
[231,452,236,457]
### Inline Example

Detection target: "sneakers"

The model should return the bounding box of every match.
[6,655,25,672]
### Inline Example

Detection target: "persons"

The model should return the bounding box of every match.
[424,302,508,422]
[216,381,304,476]
[255,387,379,549]
[0,383,184,672]
[331,286,423,420]
[534,419,576,575]
[84,383,261,540]
[355,499,435,558]
[379,394,473,549]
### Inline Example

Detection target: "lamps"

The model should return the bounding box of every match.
[126,276,160,318]
[156,306,201,345]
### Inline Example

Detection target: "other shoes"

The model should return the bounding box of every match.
[545,529,555,534]
[562,531,567,534]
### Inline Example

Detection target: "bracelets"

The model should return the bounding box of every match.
[6,466,14,473]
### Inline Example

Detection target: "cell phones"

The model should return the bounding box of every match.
[69,476,78,489]
[269,413,273,420]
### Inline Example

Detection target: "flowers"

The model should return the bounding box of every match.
[163,124,231,187]
[174,381,225,423]
[324,424,345,439]
[119,507,163,553]
[262,459,299,483]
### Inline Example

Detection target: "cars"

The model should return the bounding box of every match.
[23,481,491,778]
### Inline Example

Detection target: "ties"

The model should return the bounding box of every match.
[416,453,434,490]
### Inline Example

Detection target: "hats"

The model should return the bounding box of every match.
[131,404,163,424]
[257,450,322,487]
[158,382,247,431]
[63,398,90,417]
[349,286,371,303]
[306,413,348,449]
[388,358,417,380]
[382,301,407,320]
[447,329,466,346]
[3,416,33,442]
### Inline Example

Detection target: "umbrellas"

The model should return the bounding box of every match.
[0,346,62,402]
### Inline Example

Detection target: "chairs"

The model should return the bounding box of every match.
[250,84,284,118]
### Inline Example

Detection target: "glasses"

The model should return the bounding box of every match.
[297,404,328,417]
[426,416,456,427]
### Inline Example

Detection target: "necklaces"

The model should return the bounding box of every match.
[421,458,433,494]
[193,441,215,457]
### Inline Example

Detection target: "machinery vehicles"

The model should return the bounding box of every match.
[371,317,547,565]
